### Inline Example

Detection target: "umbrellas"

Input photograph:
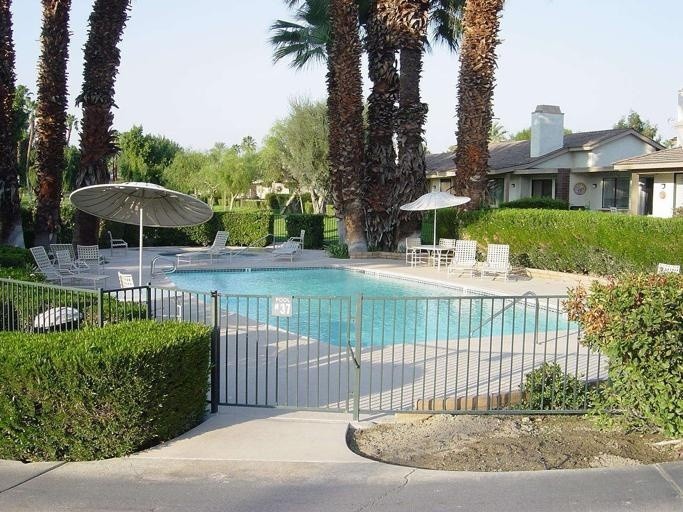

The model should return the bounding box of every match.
[399,185,471,257]
[68,181,214,287]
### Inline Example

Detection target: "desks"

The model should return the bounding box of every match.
[105,288,184,323]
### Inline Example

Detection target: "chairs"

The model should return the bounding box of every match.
[30,231,135,290]
[271,229,305,263]
[176,231,232,265]
[406,237,510,280]
[656,263,680,274]
